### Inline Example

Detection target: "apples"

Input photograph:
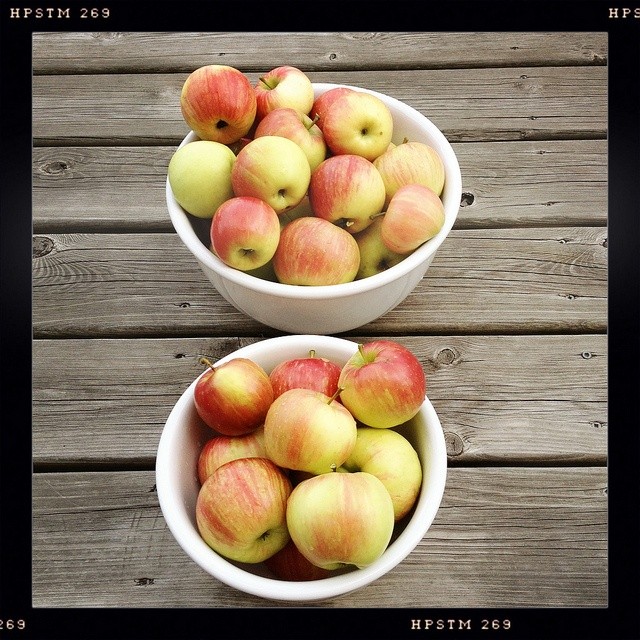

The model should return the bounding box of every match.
[352,218,405,278]
[195,457,291,564]
[230,136,311,216]
[192,357,270,438]
[337,341,426,428]
[380,182,447,255]
[342,428,422,521]
[180,65,258,144]
[372,140,444,199]
[198,434,271,484]
[253,66,314,119]
[168,141,236,218]
[323,92,393,162]
[264,388,356,474]
[309,154,386,232]
[253,107,325,171]
[273,217,361,287]
[286,472,396,569]
[210,196,280,272]
[268,357,341,400]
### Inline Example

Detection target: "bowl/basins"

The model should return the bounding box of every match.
[165,83,462,333]
[156,334,447,605]
